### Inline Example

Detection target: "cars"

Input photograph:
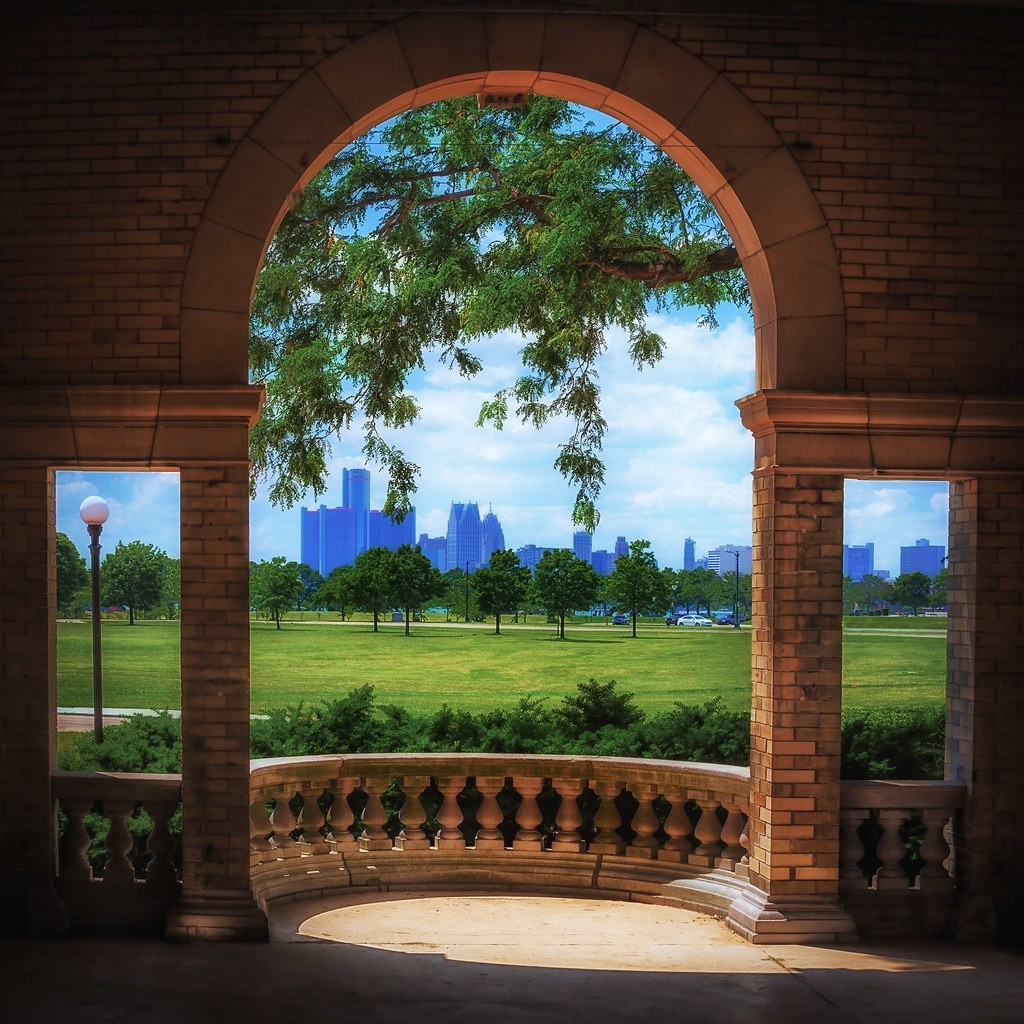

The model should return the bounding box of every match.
[612,616,629,625]
[677,615,713,627]
[83,605,122,611]
[716,614,743,625]
[664,614,686,625]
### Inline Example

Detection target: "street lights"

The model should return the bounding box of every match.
[80,495,109,744]
[724,550,741,630]
[466,558,475,622]
